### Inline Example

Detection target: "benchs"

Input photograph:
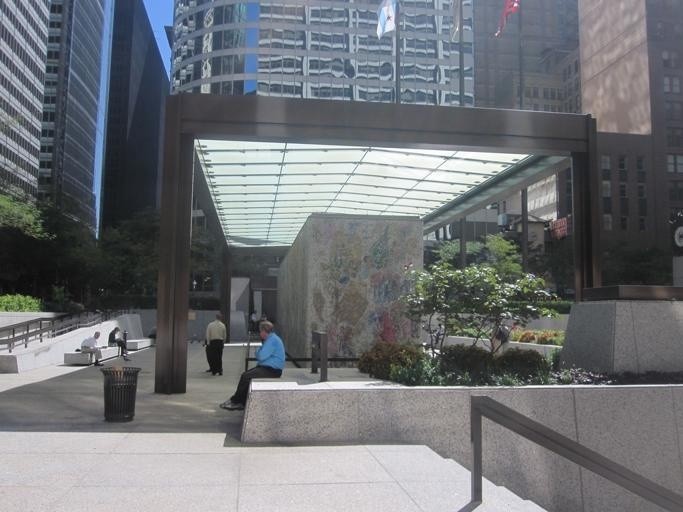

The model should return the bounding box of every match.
[63,336,154,366]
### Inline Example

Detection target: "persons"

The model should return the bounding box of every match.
[201,320,214,373]
[216,319,287,411]
[79,331,105,366]
[249,309,258,333]
[106,327,131,363]
[259,313,267,321]
[204,313,229,376]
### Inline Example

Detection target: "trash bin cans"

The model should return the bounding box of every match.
[100,367,142,422]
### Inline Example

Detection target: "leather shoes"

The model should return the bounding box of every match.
[219,399,244,411]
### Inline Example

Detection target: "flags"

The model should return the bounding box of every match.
[492,0,520,38]
[374,0,397,40]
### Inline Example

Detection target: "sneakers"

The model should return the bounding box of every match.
[206,368,222,376]
[121,354,131,361]
[94,362,104,366]
